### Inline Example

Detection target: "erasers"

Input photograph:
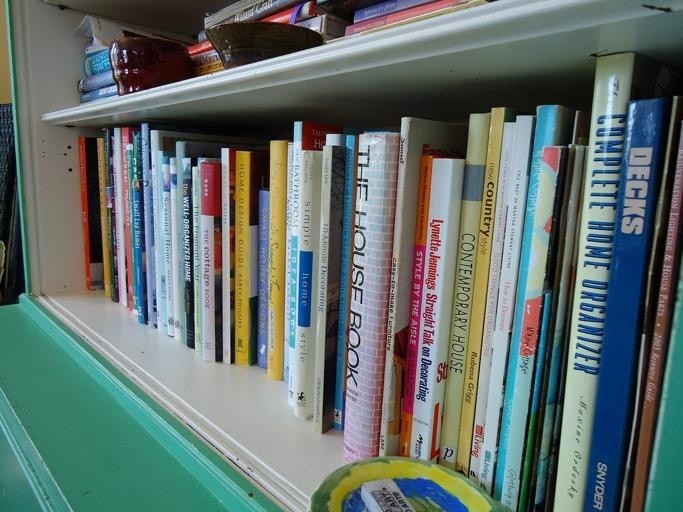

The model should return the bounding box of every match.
[361,478,417,512]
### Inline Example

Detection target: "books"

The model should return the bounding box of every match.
[75,0,484,103]
[77,52,683,510]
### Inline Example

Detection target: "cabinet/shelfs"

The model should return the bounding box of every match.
[12,4,681,512]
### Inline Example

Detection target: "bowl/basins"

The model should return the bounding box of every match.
[108,36,192,96]
[205,21,326,70]
